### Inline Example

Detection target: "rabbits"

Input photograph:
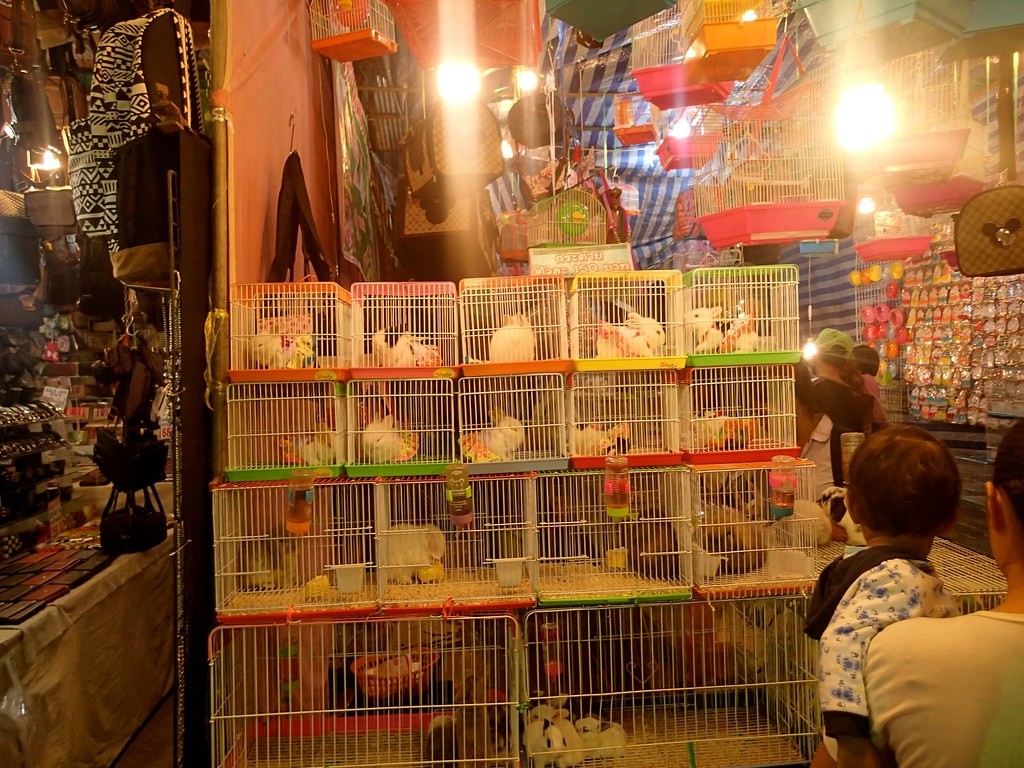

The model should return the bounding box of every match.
[234,305,868,596]
[424,674,628,768]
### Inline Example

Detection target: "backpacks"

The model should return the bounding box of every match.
[61,8,205,293]
[951,184,1024,278]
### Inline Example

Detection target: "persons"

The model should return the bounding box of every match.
[796,424,965,768]
[803,418,1022,768]
[785,327,893,544]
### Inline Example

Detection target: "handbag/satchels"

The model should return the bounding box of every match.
[93,344,169,555]
[1,4,118,405]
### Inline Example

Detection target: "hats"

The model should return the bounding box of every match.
[815,327,854,359]
